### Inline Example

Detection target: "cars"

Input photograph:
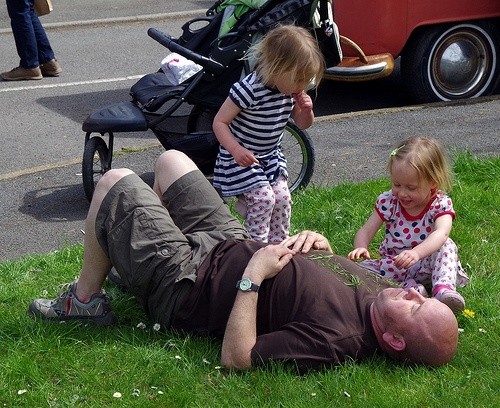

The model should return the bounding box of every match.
[323,0,500,104]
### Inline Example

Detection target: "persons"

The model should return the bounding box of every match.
[348,136,469,313]
[213,25,327,243]
[30,149,458,376]
[0,0,59,80]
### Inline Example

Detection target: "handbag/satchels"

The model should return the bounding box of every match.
[34,0,54,17]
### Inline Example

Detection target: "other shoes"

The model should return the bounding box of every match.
[435,289,465,313]
[0,66,43,80]
[39,58,63,75]
[402,279,428,299]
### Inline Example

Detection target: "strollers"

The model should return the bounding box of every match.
[82,0,343,204]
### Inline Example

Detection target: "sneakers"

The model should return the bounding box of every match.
[29,280,112,319]
[108,267,125,285]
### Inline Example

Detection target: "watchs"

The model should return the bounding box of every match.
[236,278,260,292]
